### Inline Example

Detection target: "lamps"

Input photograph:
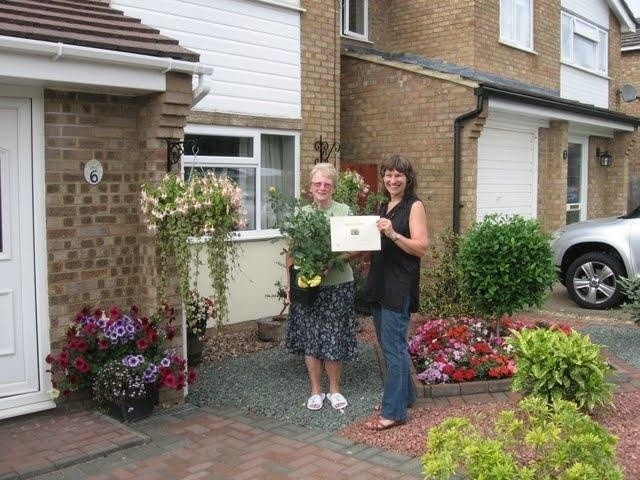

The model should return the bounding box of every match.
[596,147,613,167]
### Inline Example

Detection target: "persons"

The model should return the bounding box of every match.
[283,159,363,411]
[353,154,431,432]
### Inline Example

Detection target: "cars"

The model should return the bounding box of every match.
[547,206,640,310]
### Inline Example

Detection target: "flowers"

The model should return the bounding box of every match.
[46,287,213,425]
[266,183,346,289]
[134,166,243,331]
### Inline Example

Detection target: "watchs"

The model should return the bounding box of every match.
[393,233,401,243]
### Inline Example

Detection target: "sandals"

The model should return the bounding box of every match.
[364,415,403,429]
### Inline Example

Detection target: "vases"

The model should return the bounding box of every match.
[284,263,320,304]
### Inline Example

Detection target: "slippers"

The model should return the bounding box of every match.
[326,392,348,410]
[307,393,326,410]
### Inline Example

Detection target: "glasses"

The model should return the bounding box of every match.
[312,181,332,188]
